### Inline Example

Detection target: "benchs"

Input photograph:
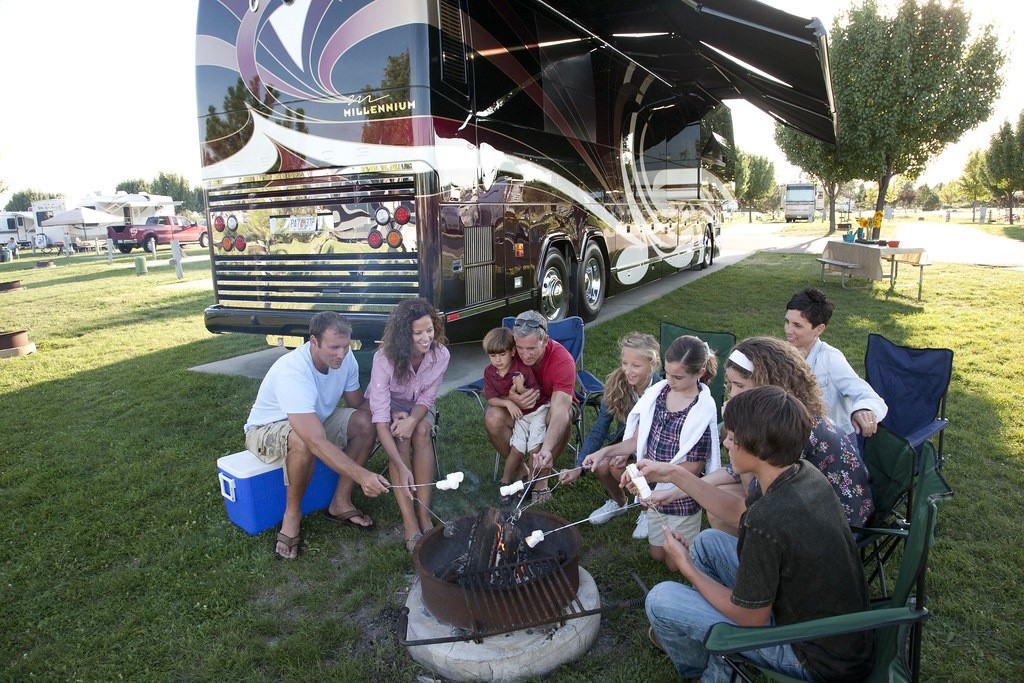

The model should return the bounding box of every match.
[885,257,937,302]
[816,257,862,290]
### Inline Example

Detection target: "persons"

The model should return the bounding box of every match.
[6,237,17,250]
[363,299,450,553]
[482,311,580,507]
[784,288,888,453]
[559,333,663,525]
[644,384,877,683]
[639,336,875,538]
[243,311,392,561]
[582,335,721,572]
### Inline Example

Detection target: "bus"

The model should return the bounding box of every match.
[195,0,839,348]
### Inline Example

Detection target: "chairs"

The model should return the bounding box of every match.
[854,421,920,597]
[346,349,447,481]
[658,321,738,424]
[457,314,607,488]
[859,334,954,512]
[704,439,956,683]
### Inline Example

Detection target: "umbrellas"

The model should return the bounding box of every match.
[41,207,124,247]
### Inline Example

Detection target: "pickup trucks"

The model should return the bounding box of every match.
[107,215,209,254]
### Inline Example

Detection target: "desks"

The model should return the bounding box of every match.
[820,238,929,293]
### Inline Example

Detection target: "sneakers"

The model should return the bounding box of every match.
[588,496,628,524]
[632,510,650,539]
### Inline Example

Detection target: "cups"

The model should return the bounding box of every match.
[877,240,886,246]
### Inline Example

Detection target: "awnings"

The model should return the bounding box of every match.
[96,198,186,214]
[541,0,838,145]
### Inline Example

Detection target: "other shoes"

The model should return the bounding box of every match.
[498,479,515,506]
[517,478,534,497]
[648,625,664,650]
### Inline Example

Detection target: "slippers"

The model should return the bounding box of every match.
[324,510,375,530]
[532,488,551,502]
[275,519,300,559]
[421,526,433,535]
[405,533,423,552]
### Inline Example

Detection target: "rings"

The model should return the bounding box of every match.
[869,422,873,423]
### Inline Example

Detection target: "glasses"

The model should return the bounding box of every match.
[514,318,546,333]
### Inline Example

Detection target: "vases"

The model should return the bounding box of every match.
[865,226,872,240]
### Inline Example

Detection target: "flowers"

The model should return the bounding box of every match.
[856,209,886,228]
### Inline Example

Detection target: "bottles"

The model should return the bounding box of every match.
[858,227,863,241]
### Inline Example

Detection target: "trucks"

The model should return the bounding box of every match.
[783,183,817,223]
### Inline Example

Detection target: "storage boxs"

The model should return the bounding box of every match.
[214,449,342,539]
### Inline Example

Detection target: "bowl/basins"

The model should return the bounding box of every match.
[888,241,900,246]
